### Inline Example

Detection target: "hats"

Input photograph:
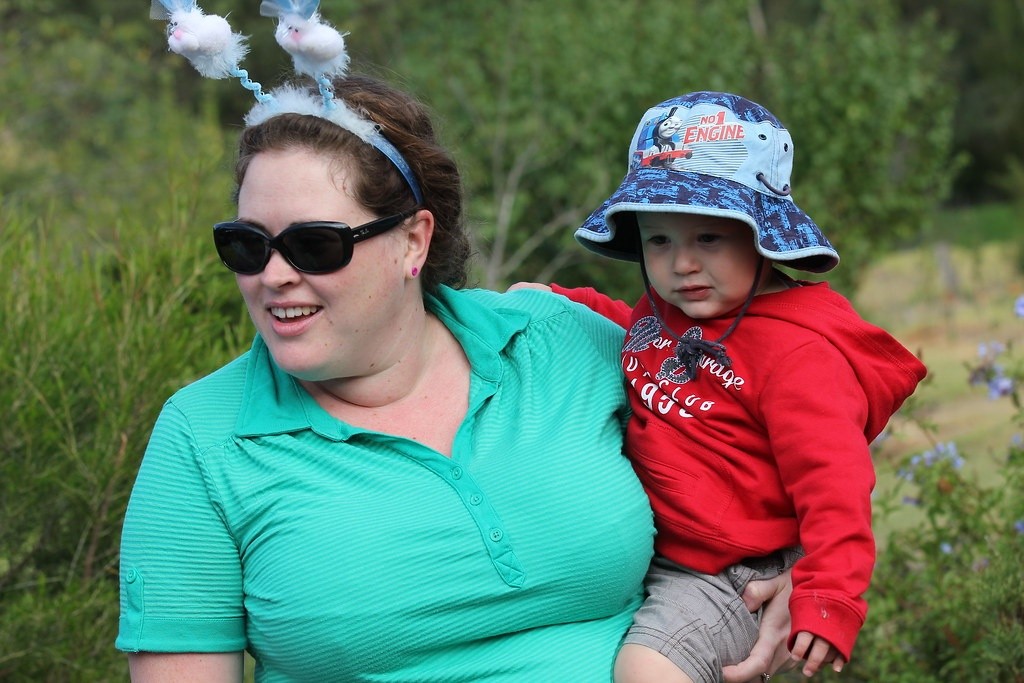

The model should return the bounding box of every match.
[574,93,839,286]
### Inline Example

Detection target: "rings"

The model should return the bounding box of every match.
[761,670,771,683]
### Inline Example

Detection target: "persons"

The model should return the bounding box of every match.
[505,89,876,683]
[117,75,810,683]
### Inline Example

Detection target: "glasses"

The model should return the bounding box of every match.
[212,207,419,279]
